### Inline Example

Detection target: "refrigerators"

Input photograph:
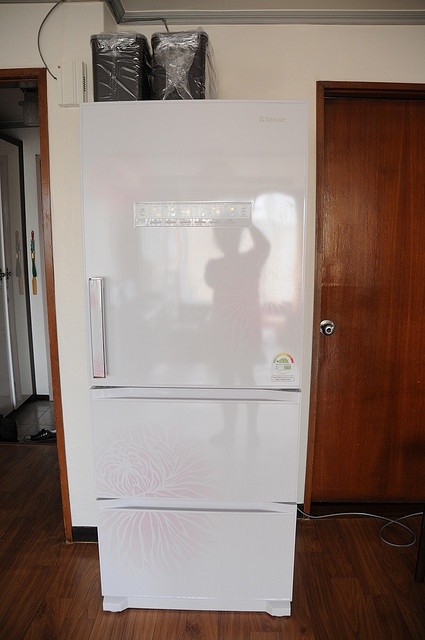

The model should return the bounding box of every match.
[80,98,317,617]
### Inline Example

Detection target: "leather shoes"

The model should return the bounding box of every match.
[23,428,57,444]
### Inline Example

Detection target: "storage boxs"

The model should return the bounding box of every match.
[90,33,152,102]
[149,30,208,100]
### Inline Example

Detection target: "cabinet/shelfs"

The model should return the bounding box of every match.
[0,139,33,420]
